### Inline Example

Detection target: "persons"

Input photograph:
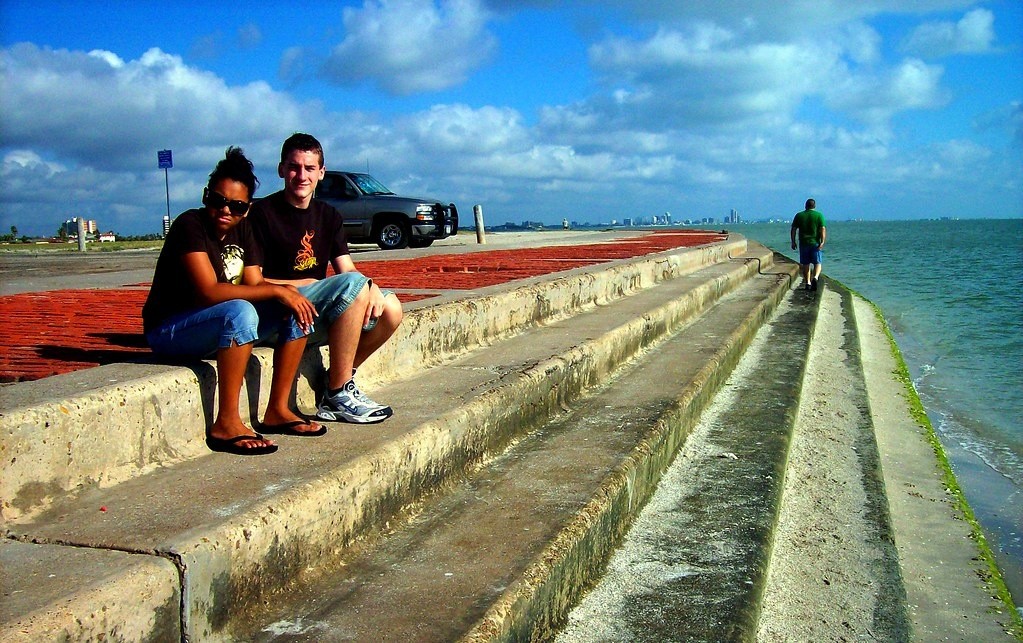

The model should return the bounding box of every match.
[791,198,826,292]
[248,132,402,423]
[142,146,328,454]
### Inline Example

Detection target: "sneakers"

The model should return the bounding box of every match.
[314,381,393,422]
[325,366,357,384]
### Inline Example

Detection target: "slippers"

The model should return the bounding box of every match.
[261,418,327,436]
[206,431,279,455]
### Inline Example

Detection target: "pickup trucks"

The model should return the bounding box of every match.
[251,170,458,250]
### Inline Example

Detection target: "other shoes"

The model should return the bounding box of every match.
[804,283,811,289]
[811,277,818,291]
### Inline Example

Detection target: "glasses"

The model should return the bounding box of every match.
[202,188,249,216]
